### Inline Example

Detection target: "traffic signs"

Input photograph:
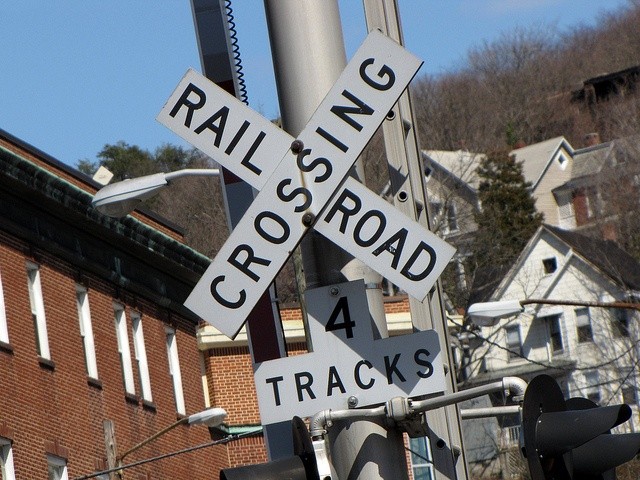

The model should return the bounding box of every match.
[220,415,319,480]
[520,374,640,479]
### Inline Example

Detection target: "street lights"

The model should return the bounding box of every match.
[467,298,640,328]
[117,407,230,463]
[93,166,219,219]
